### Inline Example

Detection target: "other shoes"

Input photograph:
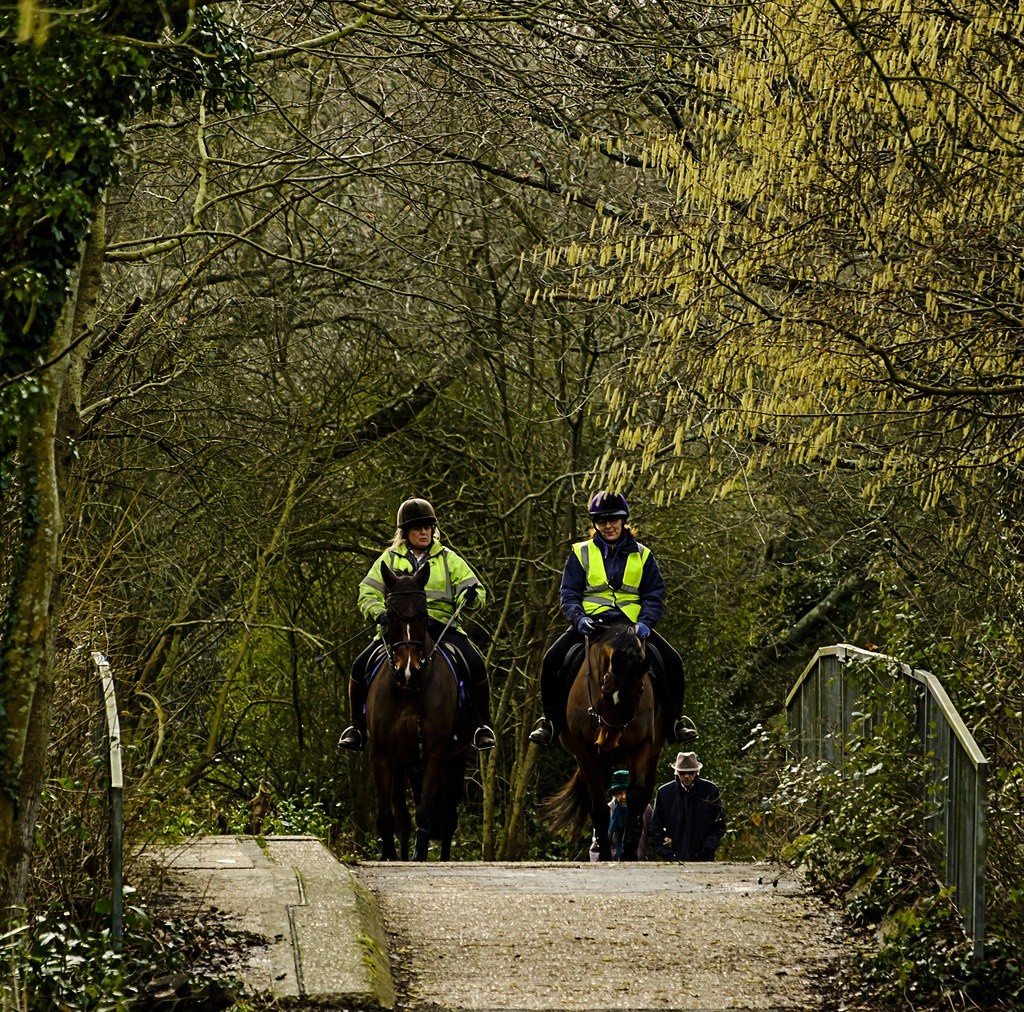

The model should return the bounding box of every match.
[339,729,369,752]
[471,729,496,750]
[666,720,700,746]
[527,721,552,744]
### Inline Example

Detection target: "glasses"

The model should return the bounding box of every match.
[595,519,622,526]
[677,771,696,776]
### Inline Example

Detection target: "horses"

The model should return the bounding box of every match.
[533,623,668,861]
[355,558,474,863]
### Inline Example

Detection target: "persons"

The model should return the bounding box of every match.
[340,497,498,753]
[529,491,702,748]
[652,753,729,862]
[590,769,674,864]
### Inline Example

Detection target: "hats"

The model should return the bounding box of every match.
[671,751,703,771]
[607,770,630,795]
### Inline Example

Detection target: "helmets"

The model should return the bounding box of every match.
[589,491,630,518]
[397,496,437,529]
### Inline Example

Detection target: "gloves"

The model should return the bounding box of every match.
[577,617,594,635]
[634,621,651,639]
[376,612,388,626]
[463,583,479,606]
